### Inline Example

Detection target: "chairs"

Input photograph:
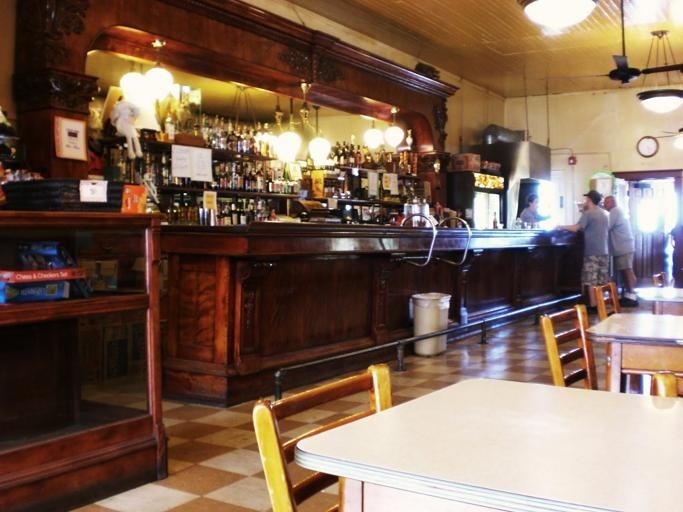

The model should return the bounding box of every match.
[249,362,392,512]
[594,280,620,321]
[539,304,598,390]
[653,272,666,288]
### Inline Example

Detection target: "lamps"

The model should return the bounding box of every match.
[363,120,384,149]
[308,105,331,163]
[119,60,144,105]
[145,41,174,103]
[636,30,683,113]
[385,112,404,148]
[277,97,301,159]
[518,0,598,29]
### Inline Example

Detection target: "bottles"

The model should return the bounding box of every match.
[318,141,420,218]
[94,113,299,225]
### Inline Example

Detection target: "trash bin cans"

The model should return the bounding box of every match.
[410,290,451,358]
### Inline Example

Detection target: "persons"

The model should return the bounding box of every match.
[602,195,637,306]
[557,191,609,313]
[516,194,548,230]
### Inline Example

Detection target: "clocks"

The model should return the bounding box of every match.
[636,136,659,158]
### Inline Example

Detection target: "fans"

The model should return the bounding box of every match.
[540,55,683,85]
[655,128,682,140]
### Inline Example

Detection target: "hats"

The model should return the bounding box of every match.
[582,190,601,201]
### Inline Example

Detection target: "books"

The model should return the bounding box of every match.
[0,268,86,283]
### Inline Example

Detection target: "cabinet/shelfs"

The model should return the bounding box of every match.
[88,113,415,224]
[1,210,168,507]
[147,224,581,408]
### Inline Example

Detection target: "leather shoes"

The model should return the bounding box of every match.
[619,297,628,302]
[586,306,598,315]
[617,299,639,307]
